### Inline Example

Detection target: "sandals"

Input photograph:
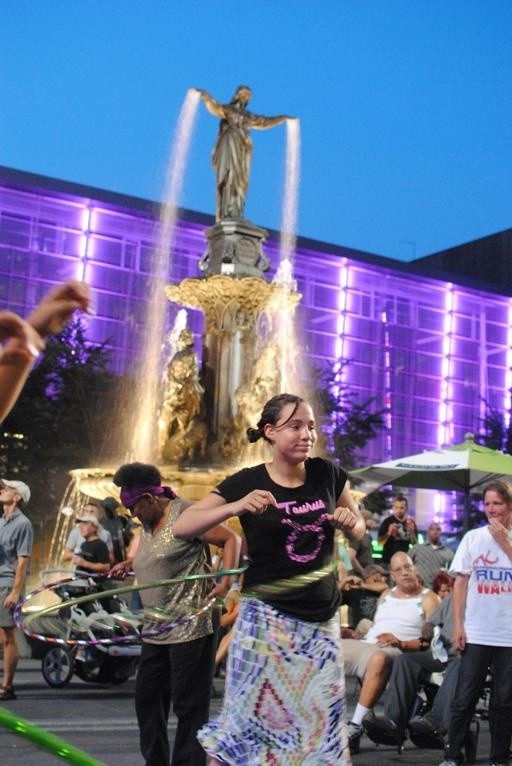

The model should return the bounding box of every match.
[0,683,15,699]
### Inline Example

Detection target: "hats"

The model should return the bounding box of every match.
[101,497,119,508]
[363,565,391,579]
[1,478,32,504]
[75,515,101,528]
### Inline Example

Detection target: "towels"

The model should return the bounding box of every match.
[430,625,448,663]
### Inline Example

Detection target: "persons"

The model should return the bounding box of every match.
[0,478,35,701]
[361,592,462,750]
[212,547,225,583]
[61,504,116,563]
[107,462,242,766]
[102,497,135,563]
[213,572,243,675]
[71,515,110,579]
[376,493,418,564]
[193,82,300,225]
[407,523,457,590]
[441,481,511,766]
[0,280,97,429]
[340,551,443,742]
[339,564,390,637]
[171,393,366,766]
[349,510,374,577]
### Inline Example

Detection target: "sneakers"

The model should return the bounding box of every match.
[408,716,444,750]
[347,722,364,741]
[362,712,401,746]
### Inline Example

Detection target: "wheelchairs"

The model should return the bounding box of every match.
[376,627,508,764]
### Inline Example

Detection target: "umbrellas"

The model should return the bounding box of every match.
[349,432,511,557]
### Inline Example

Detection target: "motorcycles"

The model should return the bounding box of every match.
[42,577,140,688]
